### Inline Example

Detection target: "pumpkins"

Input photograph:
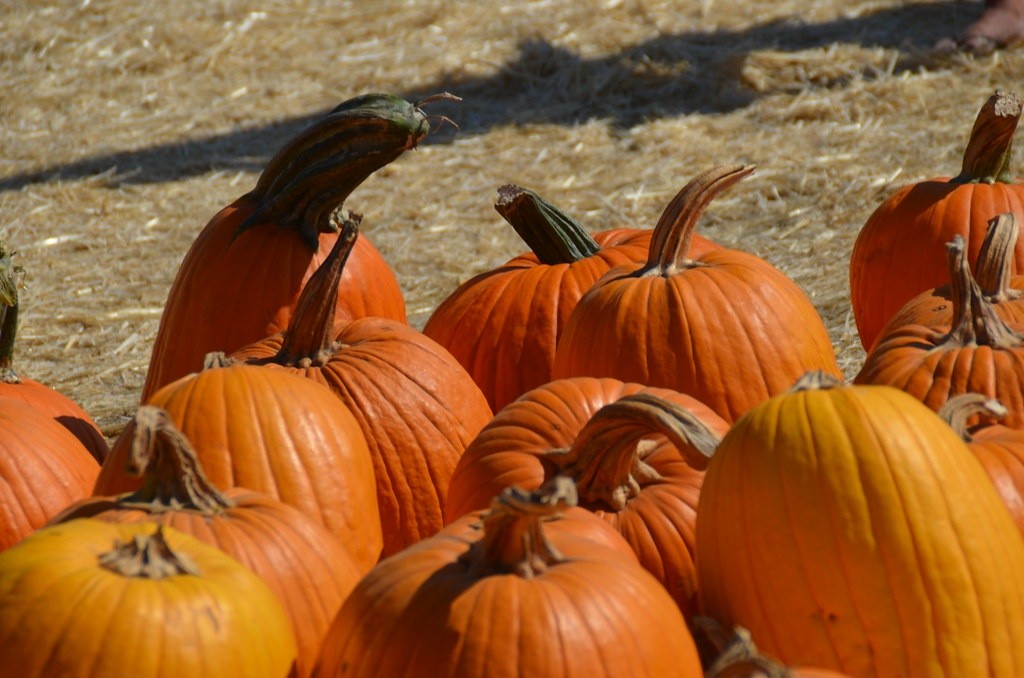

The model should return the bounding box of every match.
[0,94,1024,678]
